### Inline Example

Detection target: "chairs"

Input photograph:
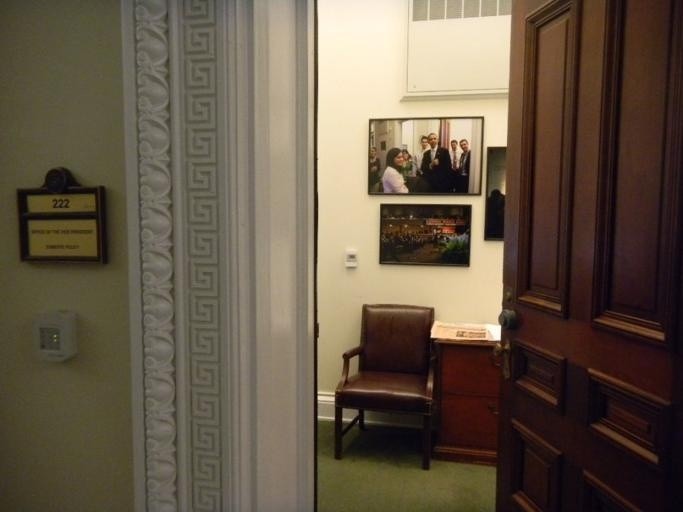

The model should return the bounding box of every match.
[329,302,441,473]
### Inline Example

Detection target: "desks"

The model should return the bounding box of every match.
[428,317,511,468]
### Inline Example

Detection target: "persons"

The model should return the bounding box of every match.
[371,132,470,193]
[380,224,469,264]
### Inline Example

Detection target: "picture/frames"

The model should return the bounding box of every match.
[375,200,476,270]
[367,115,485,199]
[484,142,508,243]
